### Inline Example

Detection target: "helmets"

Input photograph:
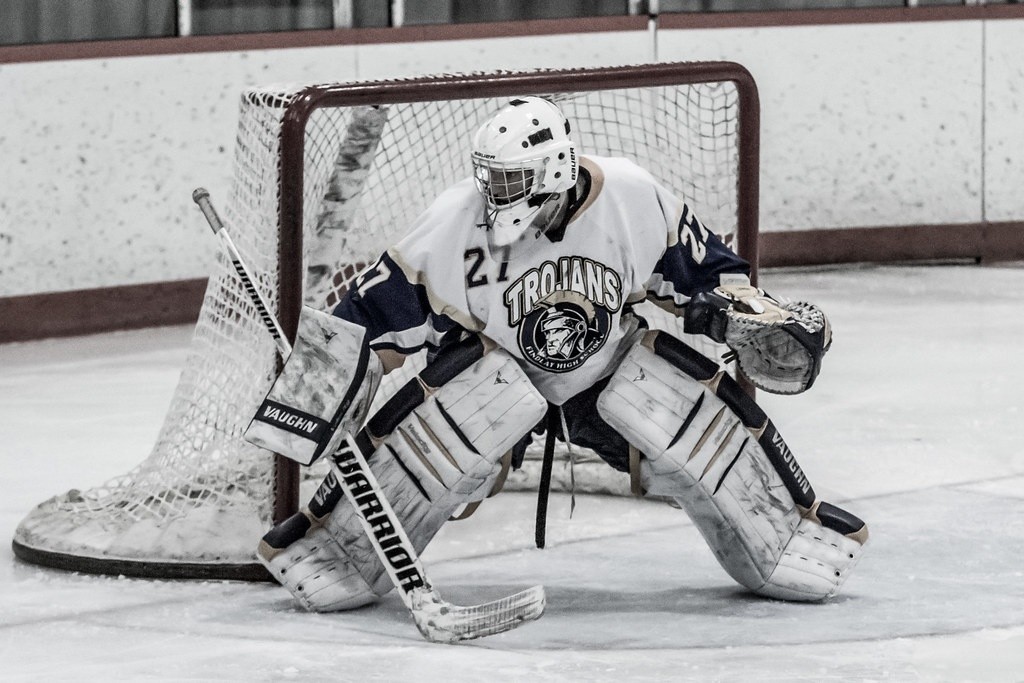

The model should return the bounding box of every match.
[473,96,578,247]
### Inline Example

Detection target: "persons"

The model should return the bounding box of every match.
[245,98,865,610]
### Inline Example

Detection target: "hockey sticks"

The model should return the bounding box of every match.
[191,187,548,645]
